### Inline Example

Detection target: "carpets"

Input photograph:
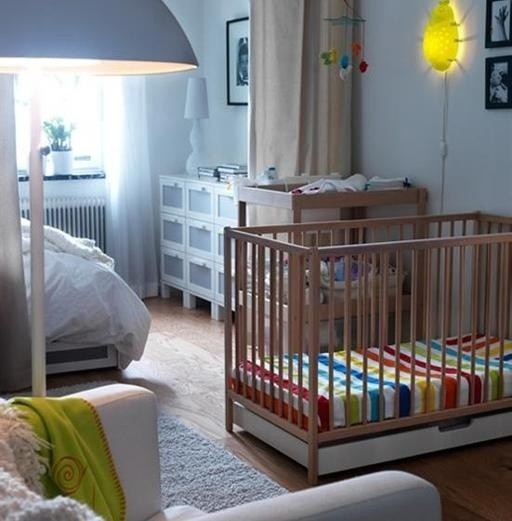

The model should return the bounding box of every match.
[3,379,290,514]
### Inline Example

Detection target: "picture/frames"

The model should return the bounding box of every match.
[485,0,511,109]
[226,18,249,106]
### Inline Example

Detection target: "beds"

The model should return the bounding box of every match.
[224,213,512,487]
[22,218,119,374]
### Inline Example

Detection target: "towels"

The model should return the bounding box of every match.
[11,395,126,521]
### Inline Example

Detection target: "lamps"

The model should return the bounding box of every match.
[1,0,200,399]
[183,77,209,174]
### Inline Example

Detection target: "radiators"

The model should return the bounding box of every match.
[20,198,107,256]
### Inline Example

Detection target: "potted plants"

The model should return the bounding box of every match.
[43,118,75,175]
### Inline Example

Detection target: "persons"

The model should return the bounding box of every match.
[238,43,248,80]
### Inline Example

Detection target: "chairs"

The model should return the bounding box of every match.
[57,384,443,521]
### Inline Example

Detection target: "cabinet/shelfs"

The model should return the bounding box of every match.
[159,176,430,321]
